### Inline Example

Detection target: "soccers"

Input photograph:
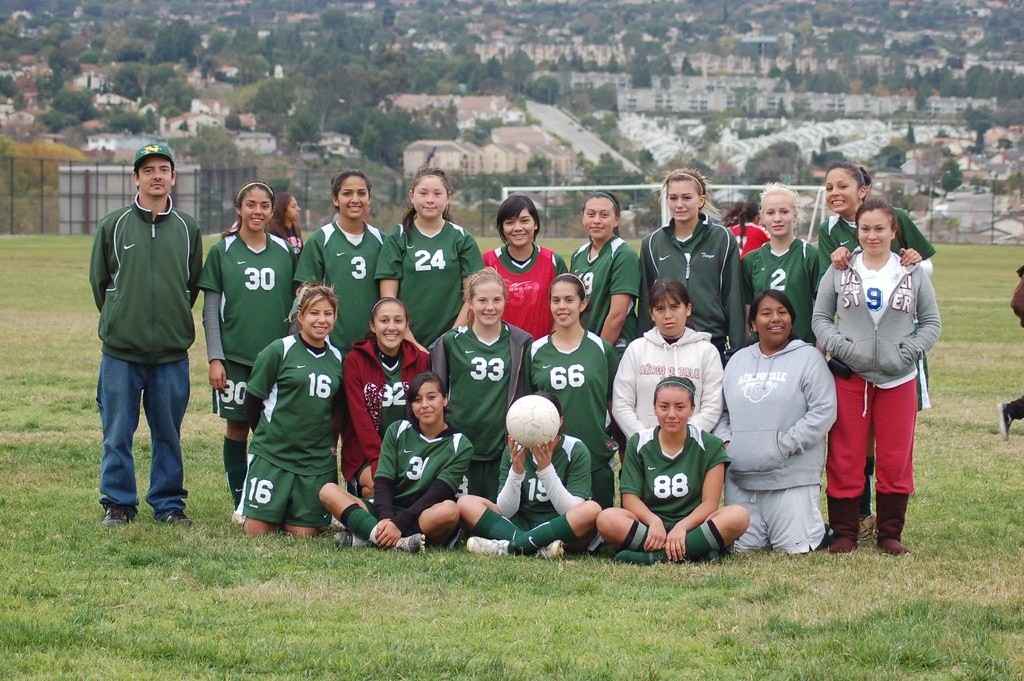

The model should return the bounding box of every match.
[506,394,559,447]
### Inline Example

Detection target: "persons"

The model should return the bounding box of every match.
[997,265,1024,440]
[293,168,387,358]
[318,371,474,554]
[522,272,620,550]
[596,376,748,565]
[376,167,484,352]
[712,289,837,554]
[812,198,941,554]
[568,189,641,349]
[482,193,567,340]
[723,201,772,259]
[457,391,603,560]
[640,167,745,366]
[340,297,432,499]
[819,162,934,539]
[239,283,343,537]
[88,144,204,528]
[196,181,296,522]
[266,192,303,262]
[737,184,819,348]
[609,278,724,441]
[428,268,533,505]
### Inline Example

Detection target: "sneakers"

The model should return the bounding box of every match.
[535,539,564,561]
[467,536,510,556]
[333,532,373,547]
[857,513,876,546]
[155,508,192,527]
[101,506,128,525]
[397,532,425,555]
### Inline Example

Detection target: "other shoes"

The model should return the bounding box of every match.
[326,515,345,529]
[824,523,833,544]
[997,402,1013,441]
[232,511,245,524]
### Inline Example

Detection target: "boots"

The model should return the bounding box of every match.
[875,490,911,556]
[826,494,861,553]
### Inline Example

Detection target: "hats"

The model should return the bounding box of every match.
[134,144,176,173]
